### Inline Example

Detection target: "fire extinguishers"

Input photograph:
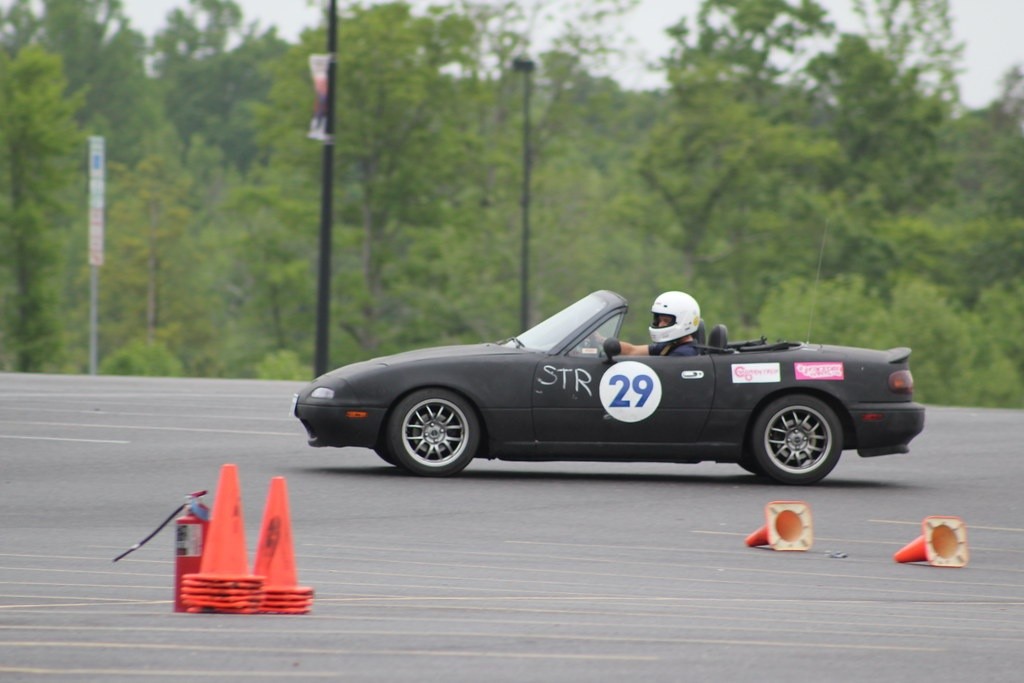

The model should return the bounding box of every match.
[112,488,211,613]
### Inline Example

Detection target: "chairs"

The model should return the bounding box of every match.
[692,319,728,356]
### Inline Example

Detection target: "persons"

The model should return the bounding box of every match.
[589,291,700,356]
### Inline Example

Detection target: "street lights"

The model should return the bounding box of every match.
[513,55,537,335]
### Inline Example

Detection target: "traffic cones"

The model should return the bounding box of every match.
[746,499,814,553]
[893,515,971,568]
[180,465,313,615]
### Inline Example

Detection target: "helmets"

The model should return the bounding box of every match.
[648,291,700,345]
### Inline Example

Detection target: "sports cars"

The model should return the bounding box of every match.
[292,284,925,486]
